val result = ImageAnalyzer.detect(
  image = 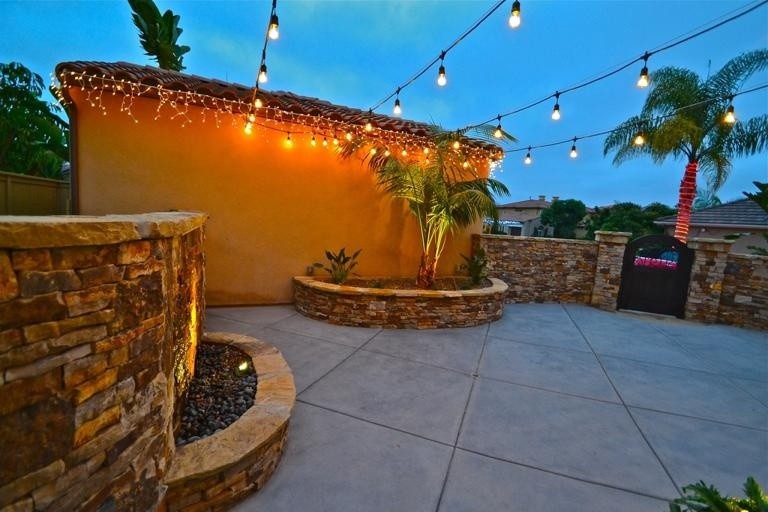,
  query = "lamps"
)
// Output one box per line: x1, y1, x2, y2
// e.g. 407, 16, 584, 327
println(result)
633, 122, 645, 146
552, 93, 560, 121
259, 50, 268, 83
267, 1, 279, 40
243, 86, 470, 170
637, 55, 649, 88
438, 54, 448, 87
525, 146, 533, 165
508, 1, 521, 28
724, 98, 735, 124
494, 118, 502, 138
570, 138, 578, 160
